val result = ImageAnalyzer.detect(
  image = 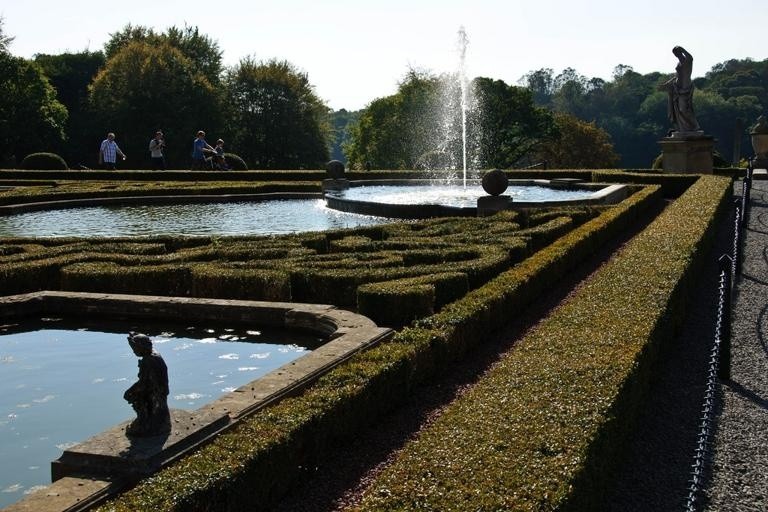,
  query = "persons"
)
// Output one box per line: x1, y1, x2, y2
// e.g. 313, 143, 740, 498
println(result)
148, 131, 167, 170
211, 138, 228, 170
122, 330, 172, 438
191, 130, 218, 170
97, 133, 127, 170
664, 46, 699, 133
